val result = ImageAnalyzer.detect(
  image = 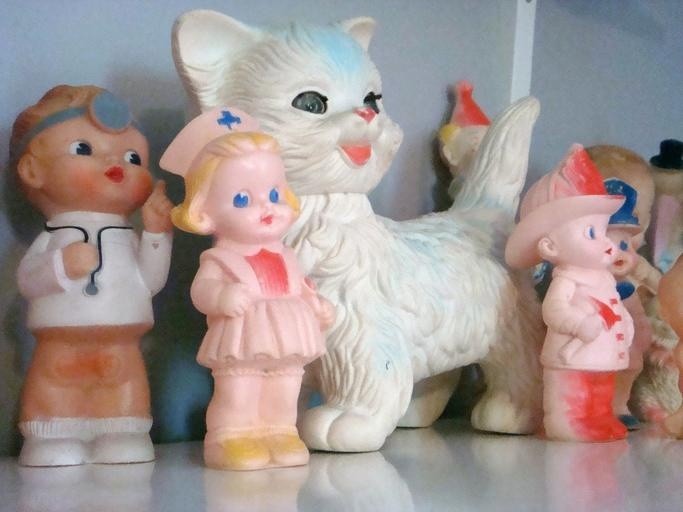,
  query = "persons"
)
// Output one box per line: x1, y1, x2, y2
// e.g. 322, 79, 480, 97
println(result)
158, 106, 338, 470
439, 80, 682, 445
7, 85, 175, 467
